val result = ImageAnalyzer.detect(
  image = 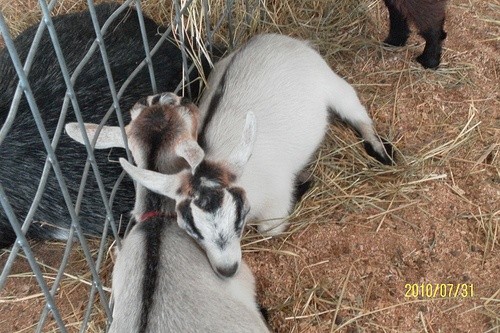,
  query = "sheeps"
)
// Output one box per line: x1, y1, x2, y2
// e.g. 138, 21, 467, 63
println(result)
64, 93, 273, 333
383, 0, 448, 71
119, 32, 393, 282
0, 1, 314, 252
